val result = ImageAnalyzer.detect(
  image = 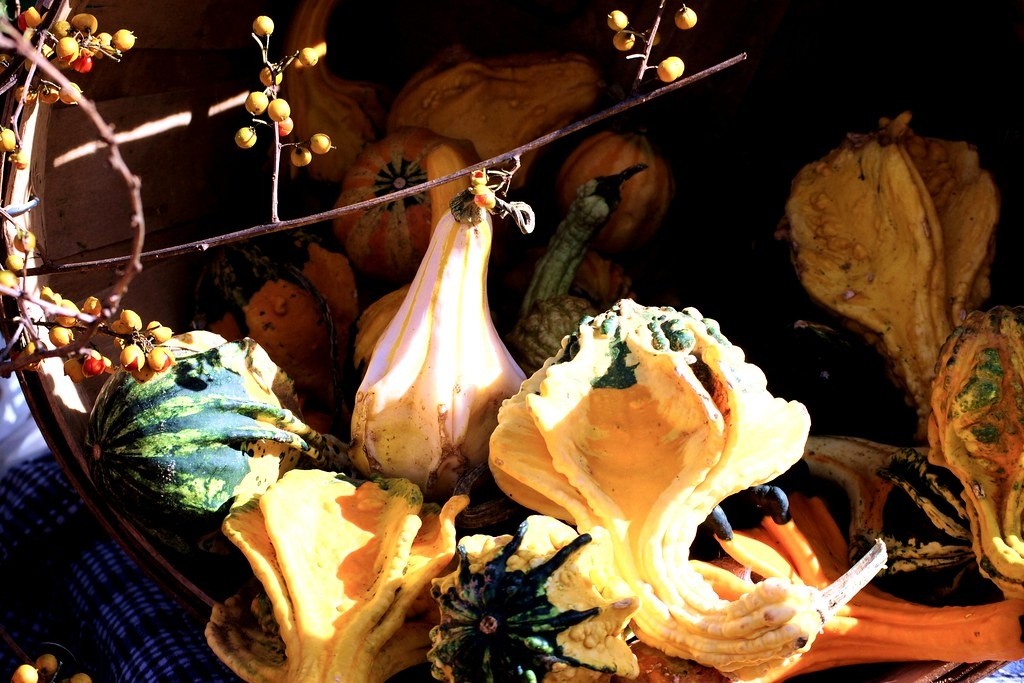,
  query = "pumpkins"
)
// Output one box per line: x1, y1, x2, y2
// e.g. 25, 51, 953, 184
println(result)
83, 8, 1023, 683
